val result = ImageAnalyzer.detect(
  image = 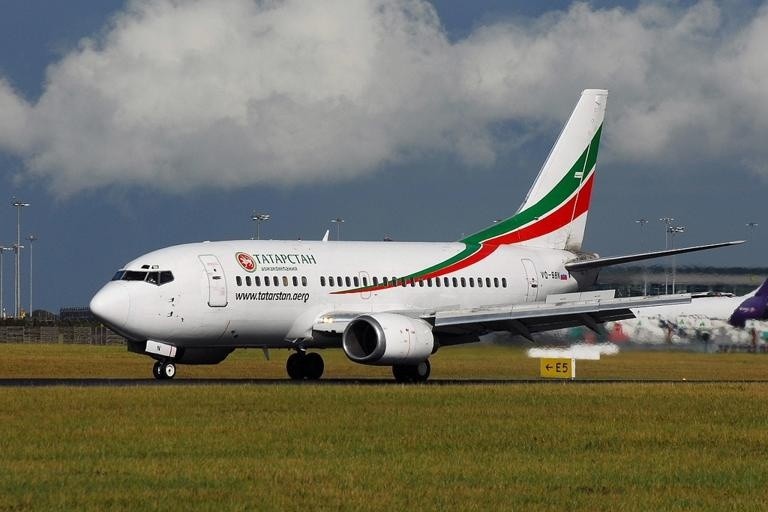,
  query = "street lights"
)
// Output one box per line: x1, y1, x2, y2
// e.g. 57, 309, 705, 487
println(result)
0, 201, 37, 320
